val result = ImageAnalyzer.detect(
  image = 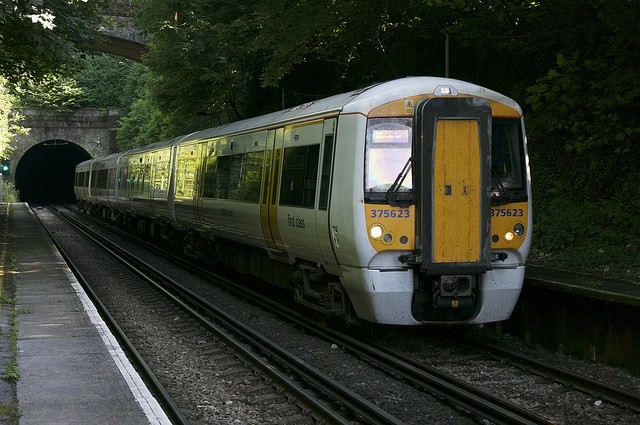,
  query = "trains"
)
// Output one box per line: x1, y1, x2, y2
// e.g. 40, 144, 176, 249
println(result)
73, 75, 533, 330
